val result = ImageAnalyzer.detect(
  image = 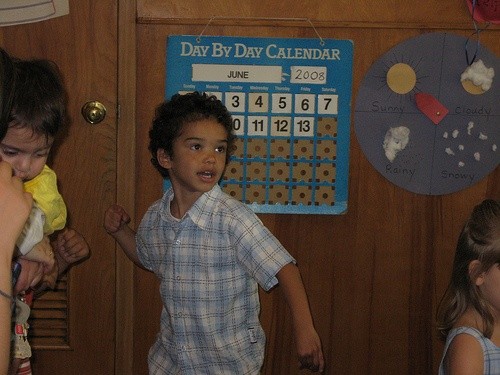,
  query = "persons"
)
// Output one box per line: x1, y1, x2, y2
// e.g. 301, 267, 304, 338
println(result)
104, 91, 324, 375
432, 198, 500, 375
0, 47, 90, 375
